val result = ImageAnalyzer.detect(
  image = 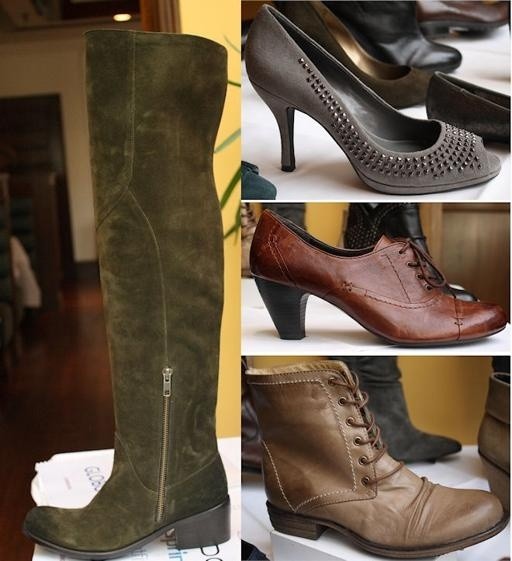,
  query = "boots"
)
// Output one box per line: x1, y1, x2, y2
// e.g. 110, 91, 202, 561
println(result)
24, 29, 230, 561
241, 356, 265, 475
329, 356, 461, 462
241, 203, 506, 346
477, 373, 510, 515
246, 360, 506, 557
